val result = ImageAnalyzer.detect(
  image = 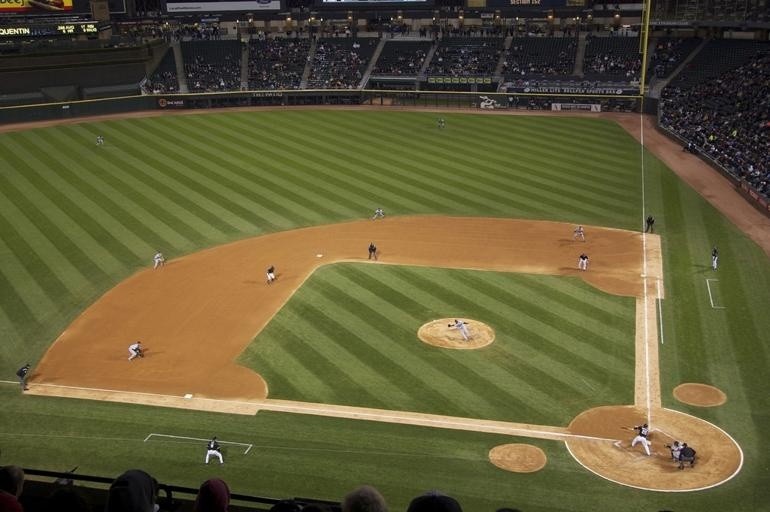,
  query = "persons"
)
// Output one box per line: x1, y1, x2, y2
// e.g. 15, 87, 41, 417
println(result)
645, 215, 654, 233
129, 341, 142, 360
16, 364, 30, 389
368, 242, 378, 260
205, 436, 224, 465
579, 253, 588, 270
574, 226, 584, 241
267, 266, 275, 284
667, 441, 680, 461
631, 424, 651, 456
711, 245, 718, 270
153, 251, 163, 270
372, 208, 385, 220
678, 443, 696, 470
449, 320, 470, 341
